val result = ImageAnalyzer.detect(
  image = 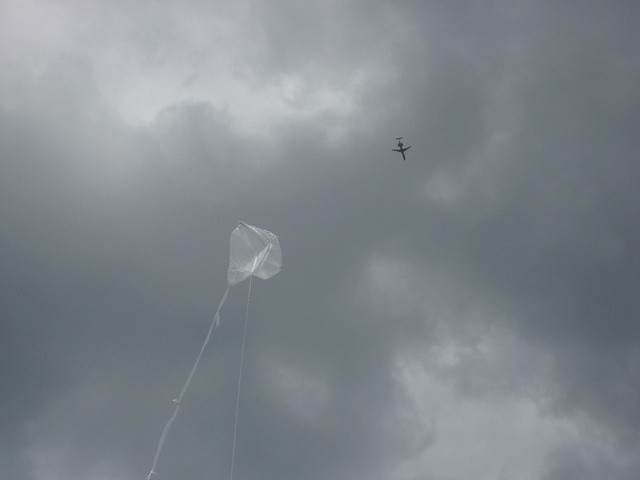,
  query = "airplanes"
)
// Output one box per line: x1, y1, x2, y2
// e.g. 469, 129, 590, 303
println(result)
391, 137, 411, 160
